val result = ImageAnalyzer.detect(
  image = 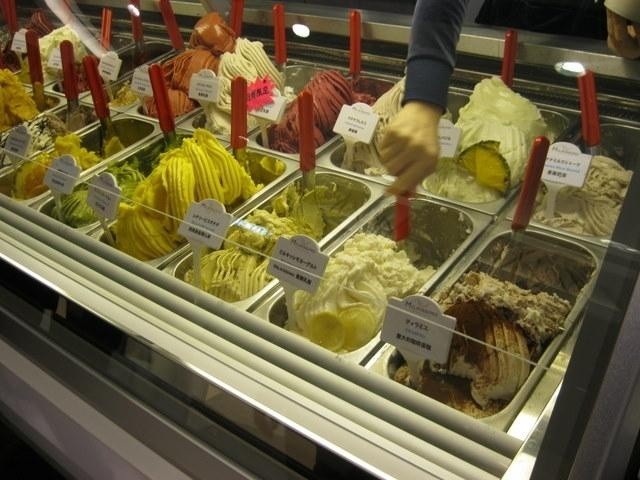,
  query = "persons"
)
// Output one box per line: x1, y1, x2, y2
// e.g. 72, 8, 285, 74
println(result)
374, 1, 640, 197
